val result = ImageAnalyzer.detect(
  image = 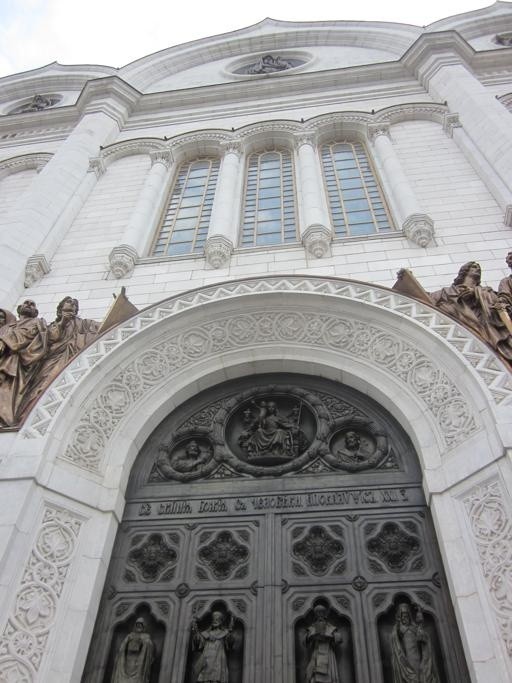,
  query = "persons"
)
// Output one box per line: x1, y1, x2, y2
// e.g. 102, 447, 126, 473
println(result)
246, 52, 294, 75
1, 294, 101, 430
172, 440, 207, 472
187, 610, 238, 682
300, 604, 344, 683
336, 431, 373, 462
493, 248, 511, 313
391, 603, 440, 682
238, 402, 298, 457
392, 257, 510, 370
108, 615, 156, 683
